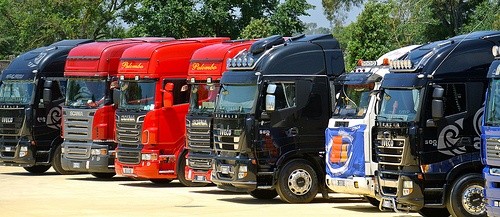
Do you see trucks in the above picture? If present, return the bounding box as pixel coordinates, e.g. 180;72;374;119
184;37;289;193
209;34;345;203
374;31;500;217
479;57;500;217
324;44;421;212
114;37;232;186
59;37;177;180
0;38;122;175
